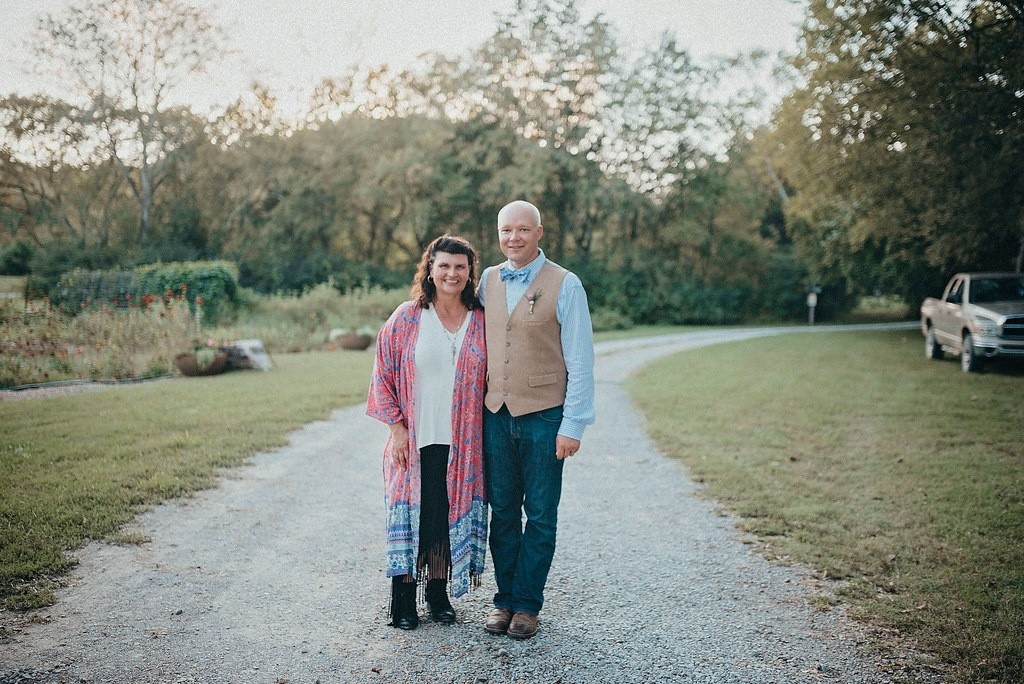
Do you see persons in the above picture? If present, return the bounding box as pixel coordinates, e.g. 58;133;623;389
475;199;595;636
365;235;490;630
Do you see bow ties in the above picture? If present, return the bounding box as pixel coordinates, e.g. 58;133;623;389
500;268;531;283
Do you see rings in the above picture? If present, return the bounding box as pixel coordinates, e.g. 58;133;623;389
393;452;399;457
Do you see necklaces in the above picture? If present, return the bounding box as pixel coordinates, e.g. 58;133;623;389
435;303;465;367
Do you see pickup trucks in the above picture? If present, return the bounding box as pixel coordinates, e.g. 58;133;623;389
919;272;1024;375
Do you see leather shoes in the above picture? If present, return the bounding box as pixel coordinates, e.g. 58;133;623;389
484;608;513;634
507;611;538;638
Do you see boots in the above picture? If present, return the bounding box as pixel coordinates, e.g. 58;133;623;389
391;575;418;629
425;578;456;622
